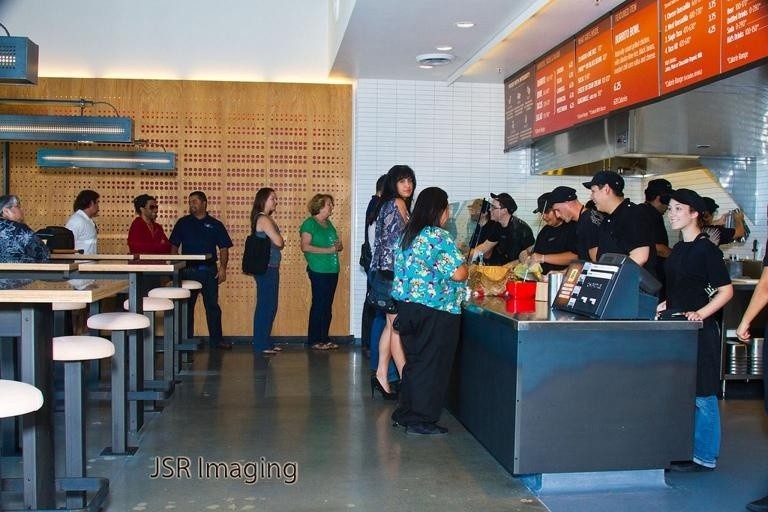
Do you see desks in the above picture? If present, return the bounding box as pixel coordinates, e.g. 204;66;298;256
46;253;137;335
78;261;186;436
139;252;213;364
0;262;79;408
0;279;130;511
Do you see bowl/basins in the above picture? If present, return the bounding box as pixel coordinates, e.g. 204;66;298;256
506;298;535;313
747;336;764;375
506;282;536;298
727;341;747;375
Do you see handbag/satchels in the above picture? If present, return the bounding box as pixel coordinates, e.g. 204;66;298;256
31;227;75;254
366;270;398;314
241;235;271;276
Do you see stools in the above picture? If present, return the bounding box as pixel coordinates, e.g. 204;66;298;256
87;312;150;458
53;337;116;512
166;280;202;363
52;303;87;335
146;287;192;380
0;379;44;419
123;297;175;412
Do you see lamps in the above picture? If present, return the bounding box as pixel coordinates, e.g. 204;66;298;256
0;36;39;85
0;114;135;143
36;147;175;170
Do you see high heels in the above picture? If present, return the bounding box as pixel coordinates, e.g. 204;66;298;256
371;374;399;401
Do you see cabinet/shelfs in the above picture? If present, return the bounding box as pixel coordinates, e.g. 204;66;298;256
715;284;768;400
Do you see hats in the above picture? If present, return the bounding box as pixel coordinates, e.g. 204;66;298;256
659;188;702;213
489;192;518;213
582;171;625;192
532;192;552;214
548;186;577;208
646;178;676;194
699;197;719;211
468;200;488;213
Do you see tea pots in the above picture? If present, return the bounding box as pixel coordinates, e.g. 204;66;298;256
728;208;740;230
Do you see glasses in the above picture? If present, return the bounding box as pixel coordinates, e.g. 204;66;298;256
144;205;158;209
490;203;503;209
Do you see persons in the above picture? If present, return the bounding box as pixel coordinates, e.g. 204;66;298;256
64;190;100;290
169;190;233;350
360;165;744;474
735;203;768;511
250;188;284;354
0;195;50;290
299;194;344;351
127;194;172;314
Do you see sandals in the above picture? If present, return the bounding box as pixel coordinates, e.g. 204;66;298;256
326;342;339;348
312;343;330;350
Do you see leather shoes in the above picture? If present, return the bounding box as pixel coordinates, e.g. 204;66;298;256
273;347;283;351
405;416;449;436
670;461;720;473
262;350;277;353
212;340;232;350
745;495;768;512
